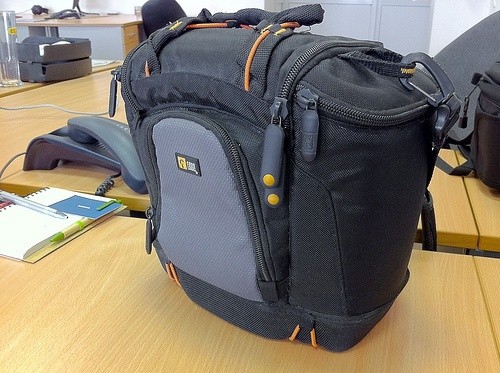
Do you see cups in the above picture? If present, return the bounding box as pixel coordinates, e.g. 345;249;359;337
0;11;21;87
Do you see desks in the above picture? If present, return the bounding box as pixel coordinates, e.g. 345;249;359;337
0;60;500;373
14;15;143;60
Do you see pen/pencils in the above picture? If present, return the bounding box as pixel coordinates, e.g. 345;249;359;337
49;199;122;243
0;190;68;220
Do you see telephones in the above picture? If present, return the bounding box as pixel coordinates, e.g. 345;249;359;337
44;9;80;19
23;113;150;194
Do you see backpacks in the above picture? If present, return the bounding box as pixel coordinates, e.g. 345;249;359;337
435;58;500;191
107;3;463;353
